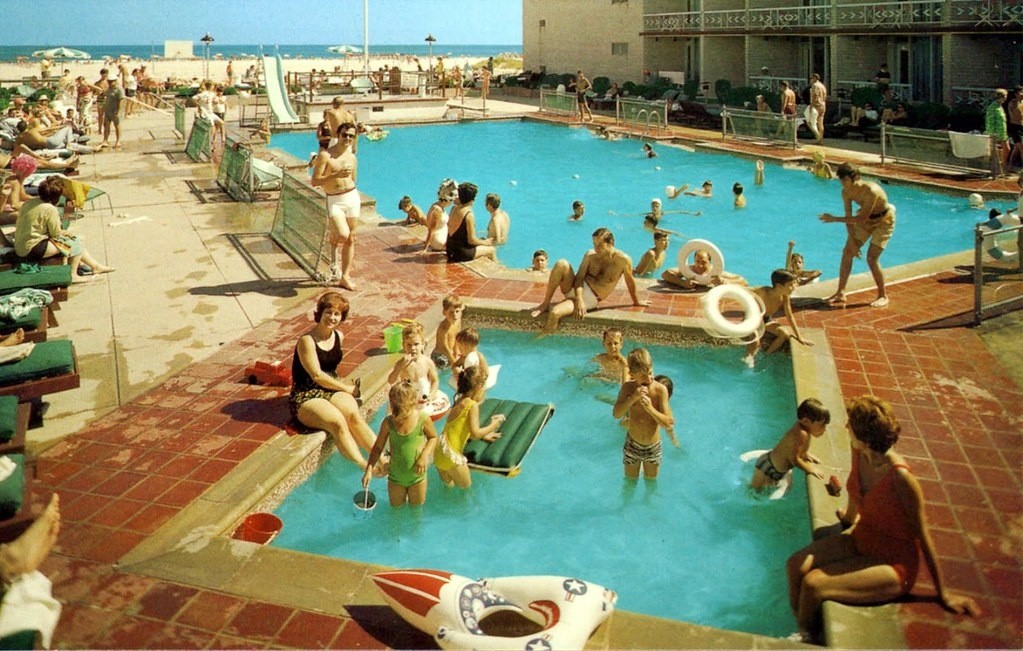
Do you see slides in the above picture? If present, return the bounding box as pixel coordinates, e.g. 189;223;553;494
262;53;301;124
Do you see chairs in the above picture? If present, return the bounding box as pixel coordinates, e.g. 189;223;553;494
503;70;911;143
0;167;80;544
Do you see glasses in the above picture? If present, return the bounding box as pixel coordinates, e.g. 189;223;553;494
340;133;354;138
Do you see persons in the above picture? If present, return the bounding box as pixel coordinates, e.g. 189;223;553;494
0;493;61;592
451;328;489;392
430;295;465;369
612;348;675;477
752;398;830;491
786;394;982;646
362;381;438;506
0;56;271;224
311;124;361;291
388;323;438;418
308;57;910;405
985;84;1023;273
14;176;116;283
433;366;502;488
289;292;391;479
0;328;25;348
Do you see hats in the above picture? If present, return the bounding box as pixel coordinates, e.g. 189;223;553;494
39;95;49;101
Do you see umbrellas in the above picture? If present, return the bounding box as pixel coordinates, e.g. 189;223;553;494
32;46;91;74
327;45;363;63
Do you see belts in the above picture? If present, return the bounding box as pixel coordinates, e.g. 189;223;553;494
870;208;890;219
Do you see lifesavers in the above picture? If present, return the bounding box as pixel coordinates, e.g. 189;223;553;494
701;284;762;337
385;388;451;422
984;214;1020;261
679;239;724;285
367;569;618;650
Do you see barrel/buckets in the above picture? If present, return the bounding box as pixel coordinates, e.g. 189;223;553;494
244;512;283;546
382;326;403;353
353;487;377;516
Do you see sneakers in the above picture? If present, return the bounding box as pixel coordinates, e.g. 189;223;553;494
77;266;92;276
